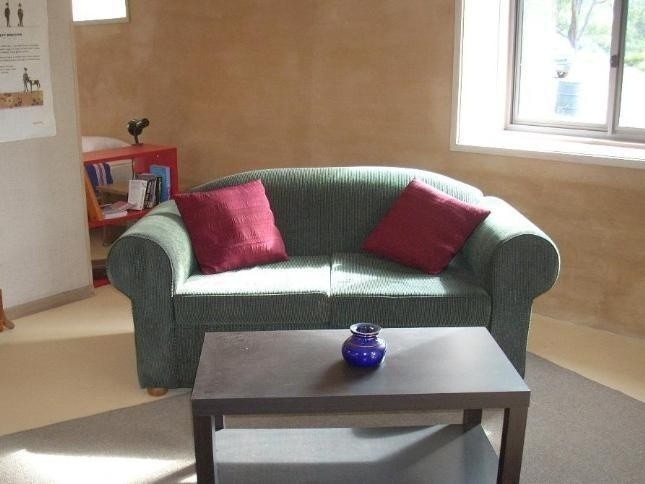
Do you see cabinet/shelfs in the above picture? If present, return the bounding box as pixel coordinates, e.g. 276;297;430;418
83;141;179;288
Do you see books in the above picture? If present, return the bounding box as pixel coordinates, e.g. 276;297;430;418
111;200;129;209
100;205;128;219
125;163;170;210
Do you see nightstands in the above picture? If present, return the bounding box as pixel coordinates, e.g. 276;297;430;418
94;183;132;246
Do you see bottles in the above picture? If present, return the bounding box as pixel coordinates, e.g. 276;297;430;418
341;323;387;367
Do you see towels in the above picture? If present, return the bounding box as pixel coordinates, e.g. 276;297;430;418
85;163;113;185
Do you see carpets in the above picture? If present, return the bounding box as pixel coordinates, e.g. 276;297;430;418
0;349;645;484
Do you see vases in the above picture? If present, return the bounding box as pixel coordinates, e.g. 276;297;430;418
341;322;386;373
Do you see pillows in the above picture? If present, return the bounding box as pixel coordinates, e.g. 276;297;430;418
171;175;290;275
355;177;492;274
82;135;132;166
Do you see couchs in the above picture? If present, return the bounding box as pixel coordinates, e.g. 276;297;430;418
103;166;560;397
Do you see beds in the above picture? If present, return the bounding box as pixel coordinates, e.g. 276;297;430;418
83;157;135;187
